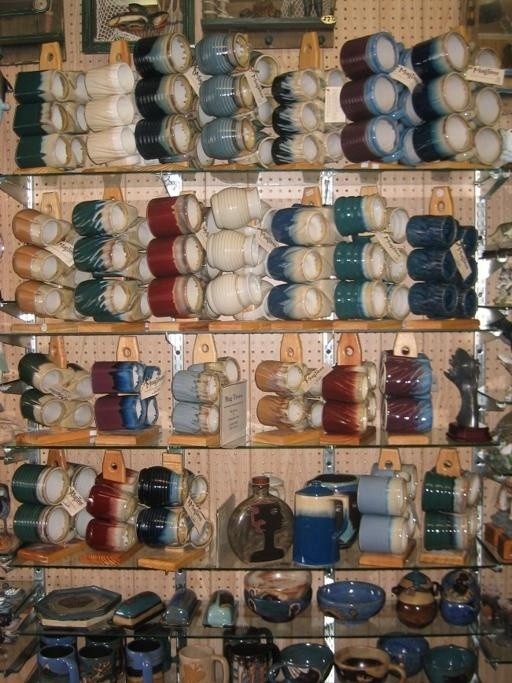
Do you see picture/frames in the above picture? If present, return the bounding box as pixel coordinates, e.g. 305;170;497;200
82;0;195;55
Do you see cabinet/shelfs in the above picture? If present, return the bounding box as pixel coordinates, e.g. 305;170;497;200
1;32;512;683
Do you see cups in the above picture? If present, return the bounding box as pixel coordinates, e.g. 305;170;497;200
453;47;504;166
12;178;484;326
16;564;486;682
12;345;485;442
11;458;483;559
11;27;486;169
